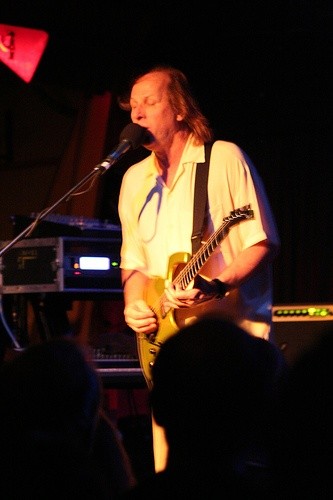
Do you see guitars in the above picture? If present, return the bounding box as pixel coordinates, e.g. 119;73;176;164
136;204;255;399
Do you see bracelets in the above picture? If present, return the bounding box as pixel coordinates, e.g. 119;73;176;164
212;279;227;299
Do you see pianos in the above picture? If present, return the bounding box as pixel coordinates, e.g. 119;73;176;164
272;294;333;323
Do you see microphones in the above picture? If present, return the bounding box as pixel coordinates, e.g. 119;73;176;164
94;123;145;175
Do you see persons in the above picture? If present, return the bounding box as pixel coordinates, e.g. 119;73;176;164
0;324;333;500
117;68;280;473
